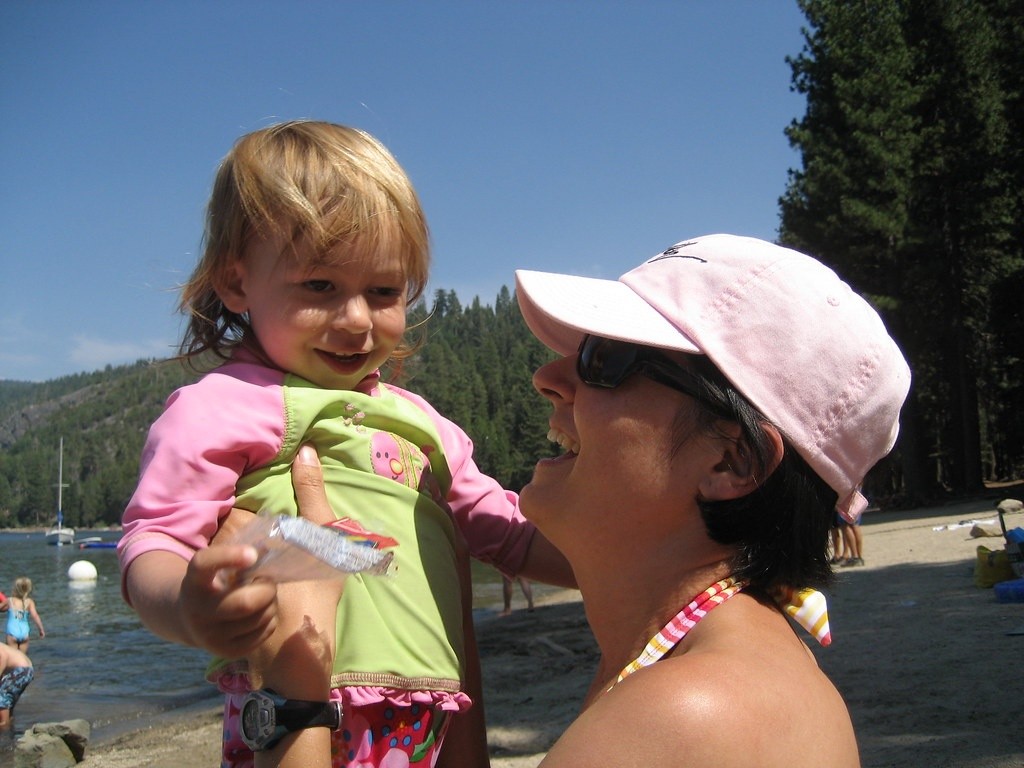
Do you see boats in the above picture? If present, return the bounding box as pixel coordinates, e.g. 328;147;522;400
74;536;117;549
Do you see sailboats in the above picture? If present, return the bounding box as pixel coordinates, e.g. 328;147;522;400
45;437;76;547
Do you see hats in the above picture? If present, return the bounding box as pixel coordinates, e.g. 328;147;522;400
513;235;912;527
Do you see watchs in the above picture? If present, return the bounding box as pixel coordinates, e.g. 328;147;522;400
238;687;344;751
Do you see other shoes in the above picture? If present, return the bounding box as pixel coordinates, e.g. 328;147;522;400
830;555;849;565
840;557;864;567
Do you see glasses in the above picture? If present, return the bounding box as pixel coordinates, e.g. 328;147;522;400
574;330;736;424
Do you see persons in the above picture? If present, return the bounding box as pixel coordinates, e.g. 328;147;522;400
116;118;582;768
210;232;911;768
828;478;865;568
0;641;34;726
0;577;45;654
497;574;534;617
